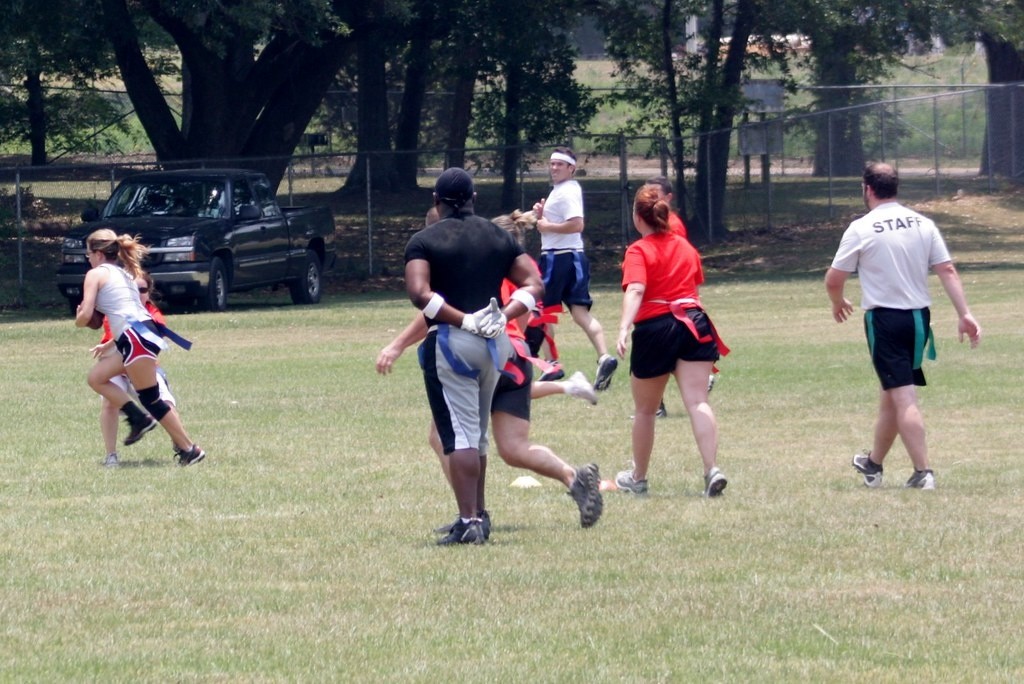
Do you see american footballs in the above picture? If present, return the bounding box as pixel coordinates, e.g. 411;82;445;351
79;301;104;329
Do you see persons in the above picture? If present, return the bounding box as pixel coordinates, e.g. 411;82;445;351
825;161;980;492
617;175;730;495
378;147;617;545
75;229;206;467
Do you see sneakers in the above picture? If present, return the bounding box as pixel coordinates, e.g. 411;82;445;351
904;469;935;491
594;352;619;389
853;454;882;488
180;444;206;467
538;359;565;382
434;509;492;539
568;371;596;406
704;468;727;497
616;469;647;496
567;463;602;529
125;415;157;446
439;519;484;547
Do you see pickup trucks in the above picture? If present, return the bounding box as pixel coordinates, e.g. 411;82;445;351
55;169;335;315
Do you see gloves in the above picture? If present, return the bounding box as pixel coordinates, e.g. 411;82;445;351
479;297;507;336
460;309;505;339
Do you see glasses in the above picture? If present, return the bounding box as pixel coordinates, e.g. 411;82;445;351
85;249;98;258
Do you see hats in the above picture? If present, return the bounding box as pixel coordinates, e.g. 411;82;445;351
434;166;475;207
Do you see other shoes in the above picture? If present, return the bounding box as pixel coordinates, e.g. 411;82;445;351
105;454;118;467
708;375;715;392
655;410;667;418
